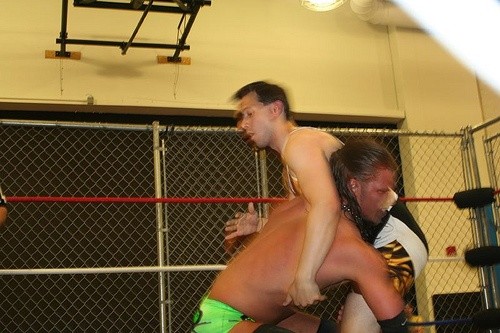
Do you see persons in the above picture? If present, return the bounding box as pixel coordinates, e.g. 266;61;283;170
194;137;408;333
224;82;428;333
403;303;425;333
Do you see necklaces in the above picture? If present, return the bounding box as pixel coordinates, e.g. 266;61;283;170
340;204;358;219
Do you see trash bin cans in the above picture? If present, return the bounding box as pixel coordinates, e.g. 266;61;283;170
432;291;485;333
